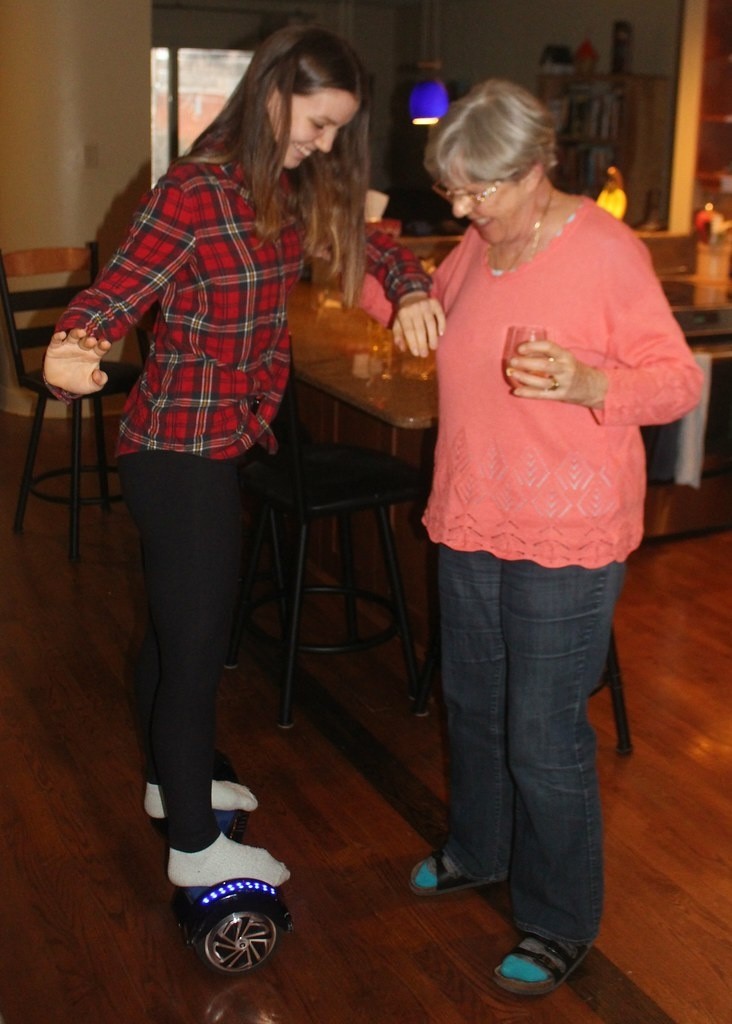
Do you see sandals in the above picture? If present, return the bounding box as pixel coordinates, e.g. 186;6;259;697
408;849;496;896
492;930;594;994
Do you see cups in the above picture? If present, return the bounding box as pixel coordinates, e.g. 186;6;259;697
696;241;732;279
502;326;549;388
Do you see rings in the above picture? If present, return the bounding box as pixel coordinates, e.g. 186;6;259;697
549;376;559;390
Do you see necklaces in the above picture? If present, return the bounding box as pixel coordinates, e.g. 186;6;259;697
483;184;553;279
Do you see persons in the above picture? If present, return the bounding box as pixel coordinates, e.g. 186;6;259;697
42;22;702;994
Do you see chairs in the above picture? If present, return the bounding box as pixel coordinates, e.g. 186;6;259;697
0;240;144;562
224;356;428;730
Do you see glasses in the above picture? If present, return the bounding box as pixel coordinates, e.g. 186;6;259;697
432;180;503;206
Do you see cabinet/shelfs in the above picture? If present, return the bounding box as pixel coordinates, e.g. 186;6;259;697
538;73;648;230
